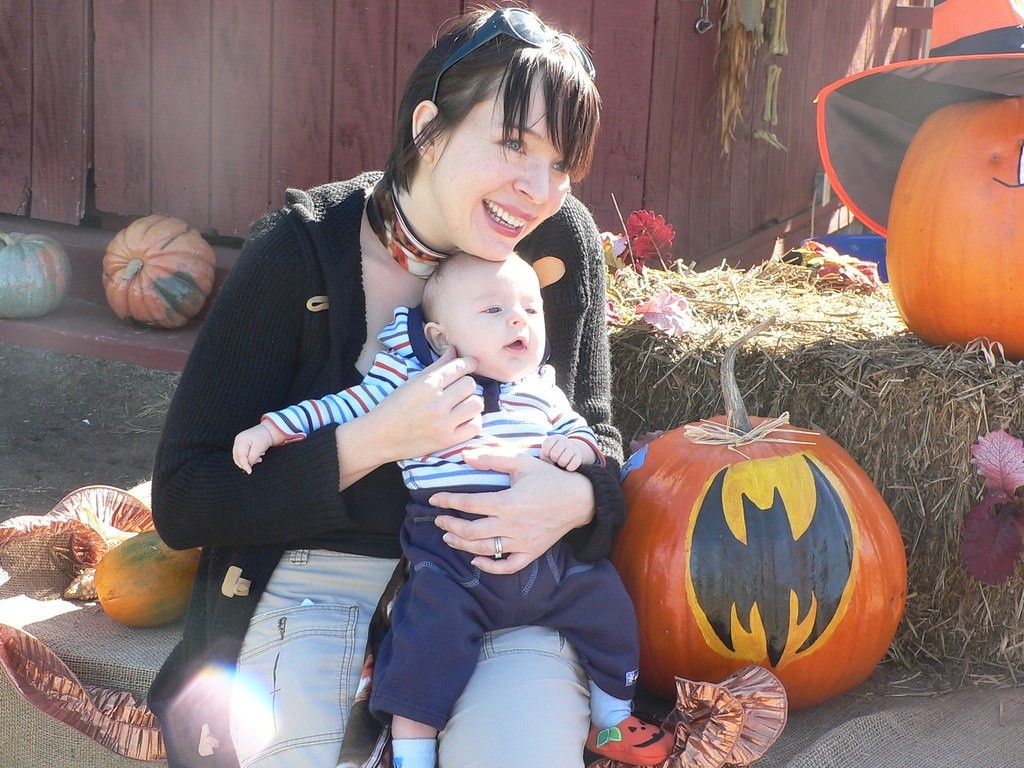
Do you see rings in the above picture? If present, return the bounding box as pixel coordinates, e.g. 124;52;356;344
494;537;503;558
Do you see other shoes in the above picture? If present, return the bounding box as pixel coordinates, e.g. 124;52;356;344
585;715;676;766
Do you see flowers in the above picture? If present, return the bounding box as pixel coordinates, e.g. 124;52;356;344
586;209;696;337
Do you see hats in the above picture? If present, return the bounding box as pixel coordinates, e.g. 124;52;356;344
818;0;1024;238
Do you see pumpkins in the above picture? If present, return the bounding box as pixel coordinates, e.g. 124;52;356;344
93;533;207;625
606;416;907;707
98;216;215;323
1;230;69;317
889;97;1024;360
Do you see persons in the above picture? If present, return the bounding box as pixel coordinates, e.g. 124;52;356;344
230;250;674;768
146;1;623;768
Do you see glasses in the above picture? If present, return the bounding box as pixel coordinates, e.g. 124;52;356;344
432;7;597;104
